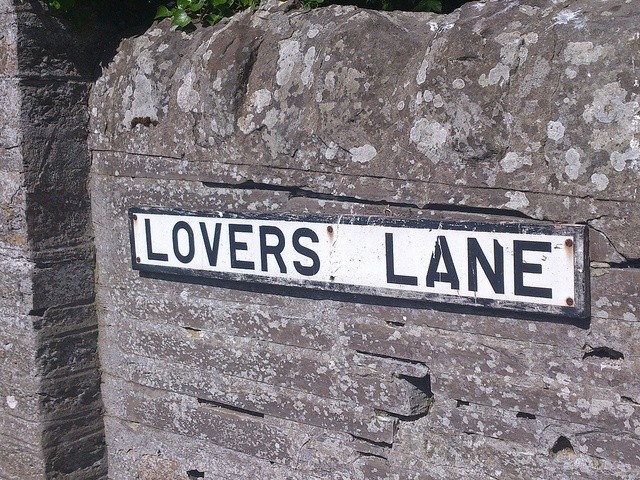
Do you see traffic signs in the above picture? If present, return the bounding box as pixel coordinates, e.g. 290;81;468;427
127;206;591;321
